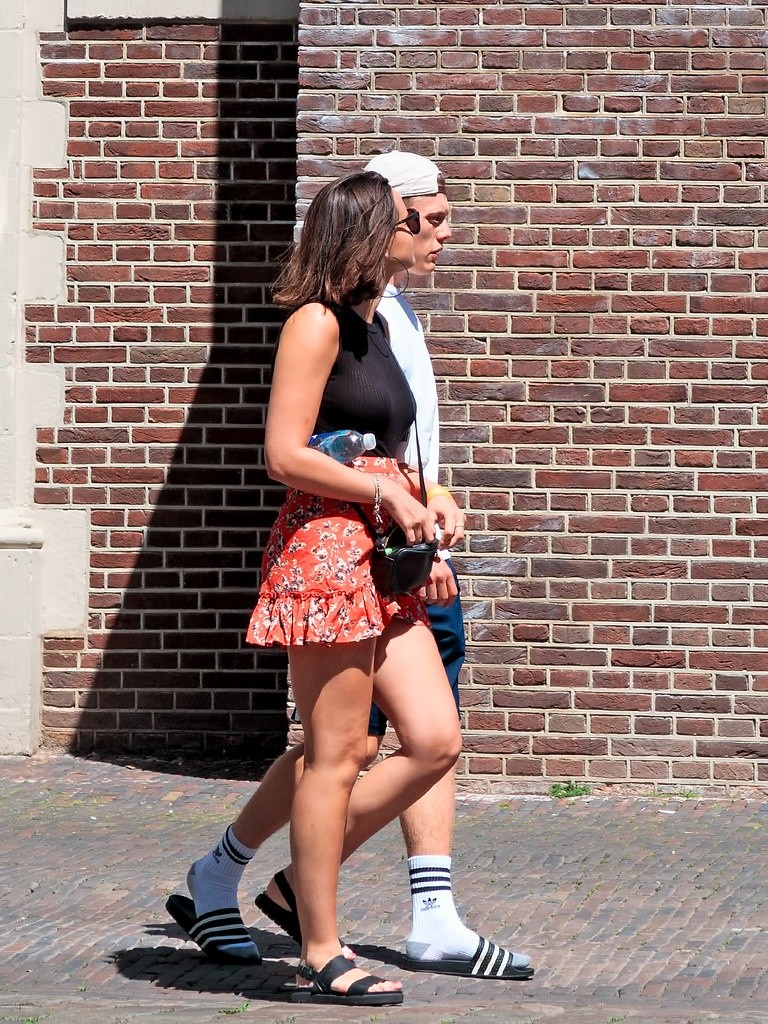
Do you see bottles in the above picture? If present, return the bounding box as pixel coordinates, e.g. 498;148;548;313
307;430;377;466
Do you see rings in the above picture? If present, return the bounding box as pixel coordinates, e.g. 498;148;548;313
456;525;464;528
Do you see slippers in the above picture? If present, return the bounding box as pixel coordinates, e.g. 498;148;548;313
401;934;536;978
165;893;262;966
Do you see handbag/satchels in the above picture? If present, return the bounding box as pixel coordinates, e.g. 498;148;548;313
371;526;437;594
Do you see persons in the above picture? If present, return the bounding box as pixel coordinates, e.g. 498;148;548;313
165;149;535;981
244;169;463;1005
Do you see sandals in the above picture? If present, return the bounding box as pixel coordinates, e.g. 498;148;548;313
255;871;357;965
291;956;404;1005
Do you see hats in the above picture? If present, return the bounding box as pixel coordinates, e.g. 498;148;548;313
362;149;447;197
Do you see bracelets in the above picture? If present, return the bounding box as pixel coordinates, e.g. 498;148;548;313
373;473;383;523
426;486;453;501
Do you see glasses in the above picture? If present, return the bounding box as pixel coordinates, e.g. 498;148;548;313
391;208;421;234
